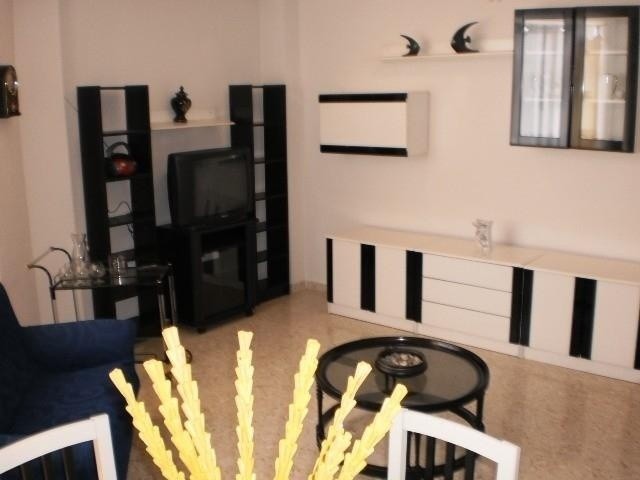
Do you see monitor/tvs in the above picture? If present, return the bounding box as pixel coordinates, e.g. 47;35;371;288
167;148;254;231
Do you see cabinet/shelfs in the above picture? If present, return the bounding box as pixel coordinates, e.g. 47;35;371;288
229;83;289;304
324;237;416;332
25;246;193;379
520;266;639;383
153;217;258;336
415;251;526;357
75;83;162;338
510;4;640;154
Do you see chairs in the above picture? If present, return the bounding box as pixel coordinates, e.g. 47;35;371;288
386;407;521;480
0;283;138;480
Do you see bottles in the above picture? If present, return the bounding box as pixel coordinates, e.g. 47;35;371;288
71;233;89;277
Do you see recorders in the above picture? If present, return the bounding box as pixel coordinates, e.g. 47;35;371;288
103;142;137;178
